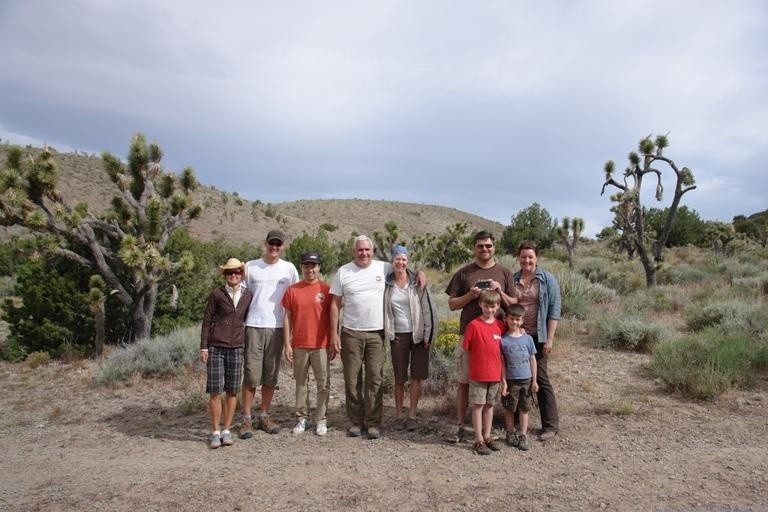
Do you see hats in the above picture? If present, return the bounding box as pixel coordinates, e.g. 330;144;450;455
301;252;322;264
267;230;283;243
220;258;245;275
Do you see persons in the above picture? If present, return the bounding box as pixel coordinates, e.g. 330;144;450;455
328;234;426;440
461;288;527;455
498;303;539;451
280;252;338;436
444;230;519;443
384;244;438;432
511;239;562;441
199;256;253;448
239;230;301;439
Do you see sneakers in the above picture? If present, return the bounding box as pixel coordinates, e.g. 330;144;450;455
259;415;279;434
519;434;529;450
447;424;464;443
239;418;253;439
395;416;407;430
407;417;416;431
350;424;364;436
540;431;558;440
293;419;312;434
507;431;519;447
367;424;380;438
316;419;327;436
473;442;491;455
484;437;502;451
222;434;232;445
211;435;221;448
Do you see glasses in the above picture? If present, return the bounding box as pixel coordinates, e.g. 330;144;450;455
224;270;242;275
475;244;494;249
269;241;282;246
